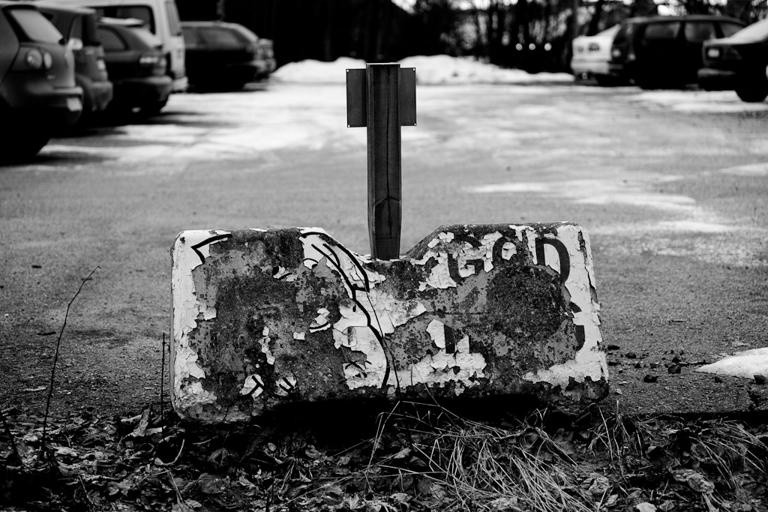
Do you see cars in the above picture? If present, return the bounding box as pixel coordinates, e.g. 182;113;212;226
694;19;768;102
180;20;277;93
569;22;652;87
0;1;188;166
608;13;752;90
